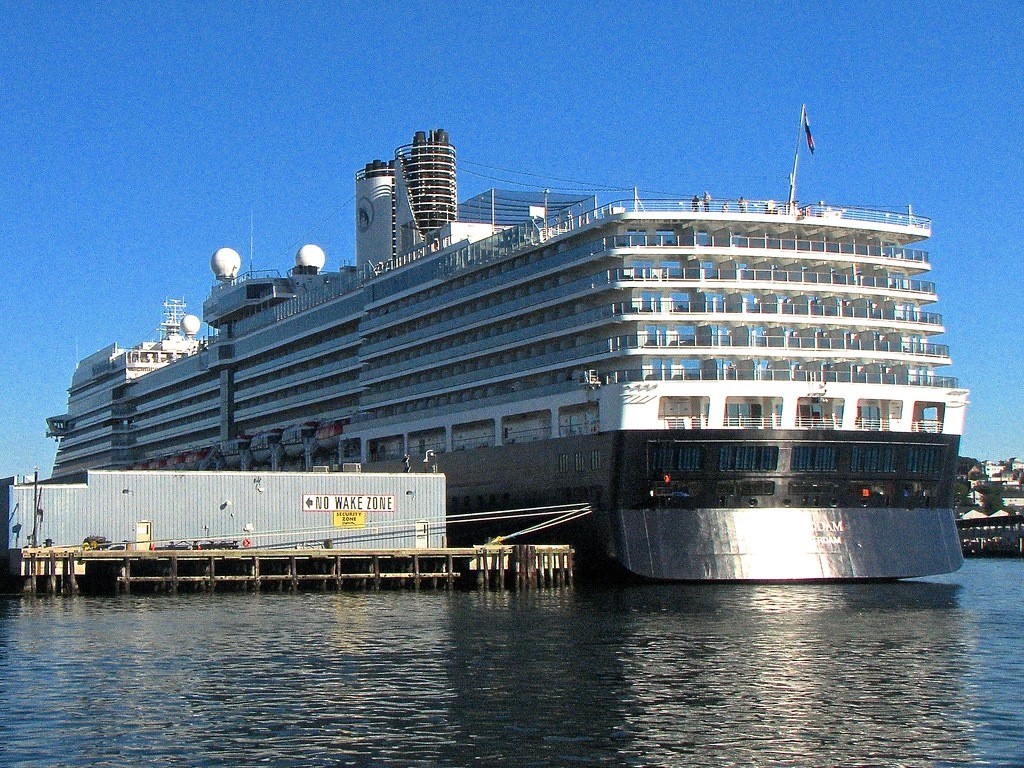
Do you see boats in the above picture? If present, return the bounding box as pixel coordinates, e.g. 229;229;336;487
148;456;168;471
185;447;209;470
250;427;284;462
315;418;347;448
281;423;320;458
221;433;254;466
165;451;188;470
121;465;133;471
132;462;149;471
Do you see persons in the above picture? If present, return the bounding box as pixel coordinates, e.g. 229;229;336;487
703;191;710;212
692;195;700;212
721;202;729;212
738;197;746;213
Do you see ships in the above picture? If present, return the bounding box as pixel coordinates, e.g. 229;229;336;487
48;100;969;586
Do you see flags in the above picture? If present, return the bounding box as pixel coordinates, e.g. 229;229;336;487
801;107;815;155
